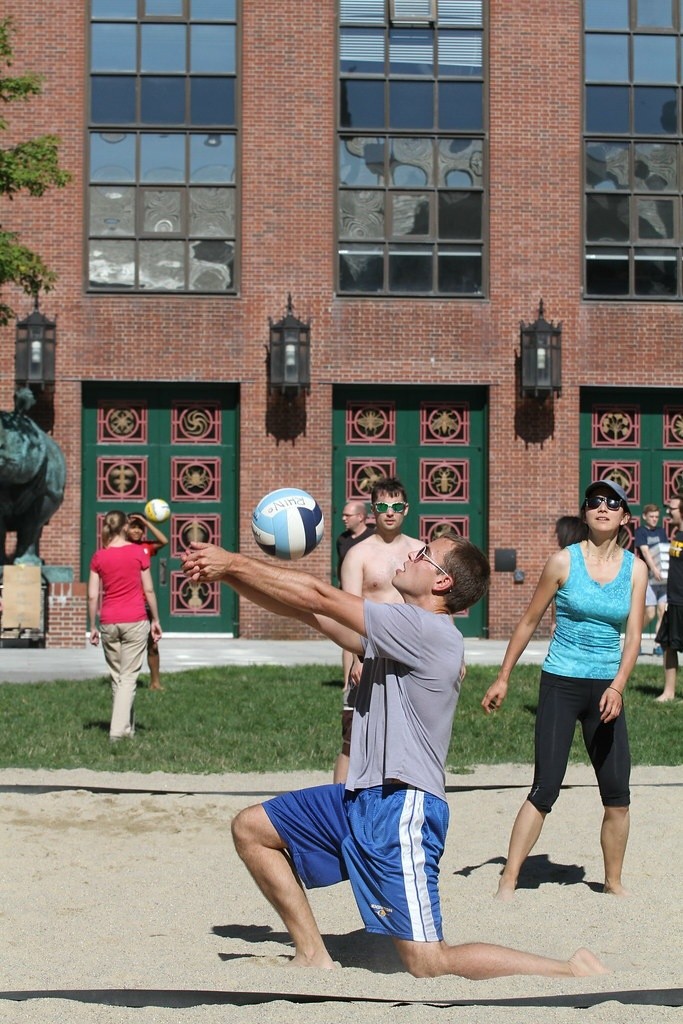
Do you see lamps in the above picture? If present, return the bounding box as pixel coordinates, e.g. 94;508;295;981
519;298;564;405
266;291;312;394
11;297;59;393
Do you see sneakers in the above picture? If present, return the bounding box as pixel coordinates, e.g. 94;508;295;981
653;644;664;658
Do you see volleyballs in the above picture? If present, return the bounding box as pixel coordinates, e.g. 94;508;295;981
251;487;326;561
144;498;171;523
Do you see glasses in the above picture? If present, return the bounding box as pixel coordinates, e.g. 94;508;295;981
374;502;406;513
584;497;623;511
415;546;453;593
342;513;360;519
668;506;679;512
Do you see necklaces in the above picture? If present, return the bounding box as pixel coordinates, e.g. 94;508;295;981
587;544;619;560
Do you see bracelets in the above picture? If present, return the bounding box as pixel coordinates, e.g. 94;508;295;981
609;687;622;696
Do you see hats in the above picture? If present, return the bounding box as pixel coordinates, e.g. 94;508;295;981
581;479;632;516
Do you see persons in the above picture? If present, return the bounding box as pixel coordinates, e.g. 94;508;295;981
179;534;605;981
336;501;377;692
333;477;465;783
88;510;162;740
482;479;648;896
653;495;683;701
126;513;168;688
634;504;667;656
